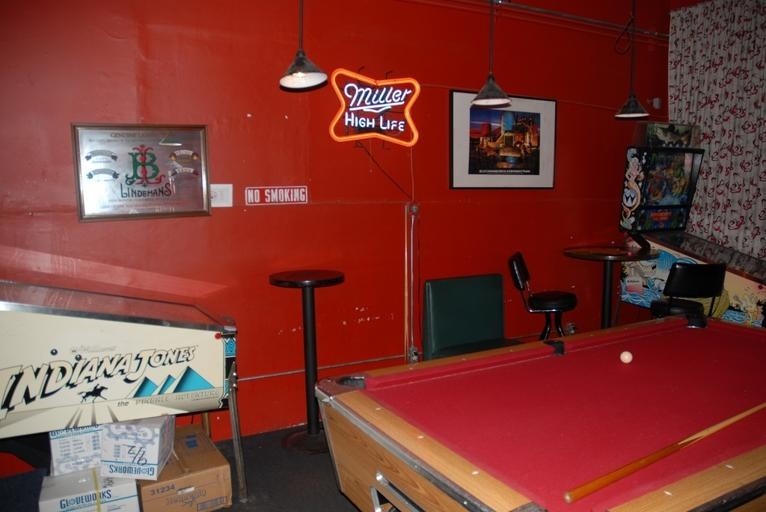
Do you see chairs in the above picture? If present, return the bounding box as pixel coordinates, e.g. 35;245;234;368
649;260;732;330
503;249;579;344
417;271;524;359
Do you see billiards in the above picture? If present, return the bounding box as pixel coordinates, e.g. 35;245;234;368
621;351;633;363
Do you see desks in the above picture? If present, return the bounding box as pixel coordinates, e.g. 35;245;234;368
265;261;344;452
562;234;661;335
312;313;765;512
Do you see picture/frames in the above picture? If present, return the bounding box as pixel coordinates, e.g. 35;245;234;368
73;120;216;224
448;93;562;193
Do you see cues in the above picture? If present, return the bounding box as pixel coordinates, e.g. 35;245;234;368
564;401;766;502
404;205;408;361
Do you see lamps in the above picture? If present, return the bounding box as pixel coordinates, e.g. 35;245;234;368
613;1;653;124
274;1;332;94
469;0;517;110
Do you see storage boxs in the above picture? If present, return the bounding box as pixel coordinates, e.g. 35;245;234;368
34;407;236;512
